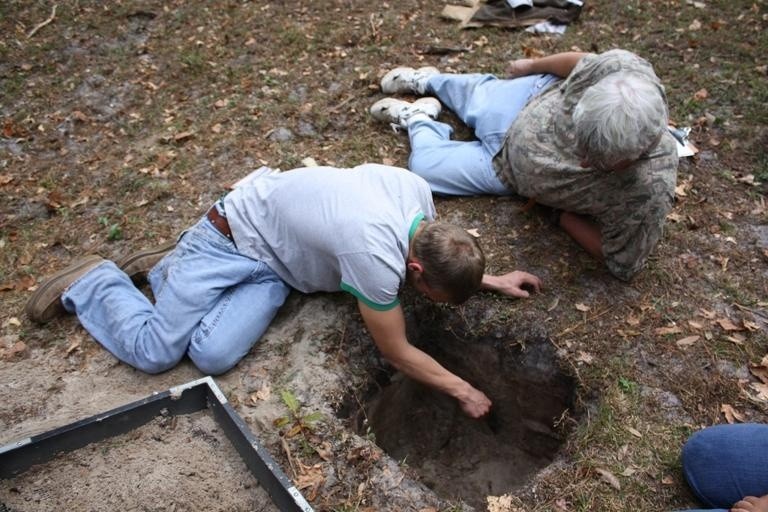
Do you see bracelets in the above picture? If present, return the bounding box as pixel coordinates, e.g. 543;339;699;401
550;208;567;222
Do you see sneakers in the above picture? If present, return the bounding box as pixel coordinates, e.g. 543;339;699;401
117;239;176;284
27;255;104;325
379;65;441;96
370;97;442;128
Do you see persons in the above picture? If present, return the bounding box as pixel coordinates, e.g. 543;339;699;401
681;425;768;512
24;160;546;420
368;49;682;284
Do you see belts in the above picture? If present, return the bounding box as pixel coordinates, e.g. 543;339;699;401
207;205;232;243
